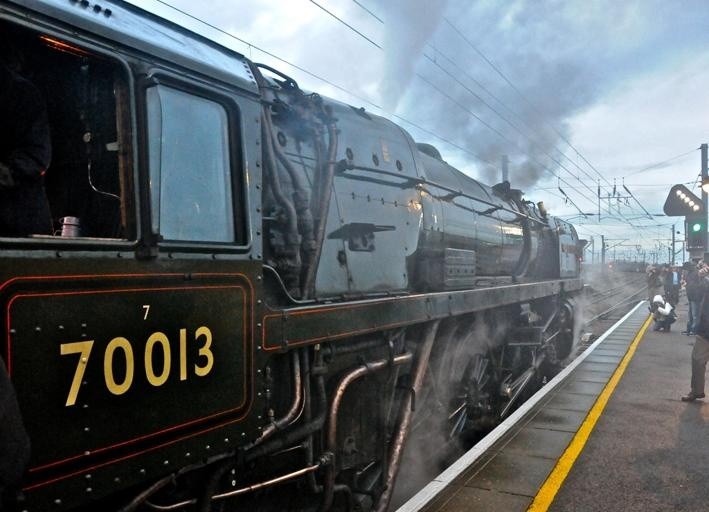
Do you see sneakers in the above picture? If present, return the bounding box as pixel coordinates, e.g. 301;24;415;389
682;391;706;403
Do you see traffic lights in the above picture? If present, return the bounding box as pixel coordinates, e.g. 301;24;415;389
687;219;706;249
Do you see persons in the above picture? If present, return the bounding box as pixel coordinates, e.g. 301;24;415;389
681;265;709;402
645;256;709;335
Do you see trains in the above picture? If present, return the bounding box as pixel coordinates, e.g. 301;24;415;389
0;0;588;510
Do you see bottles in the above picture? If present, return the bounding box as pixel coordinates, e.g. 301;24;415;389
60;216;79;237
